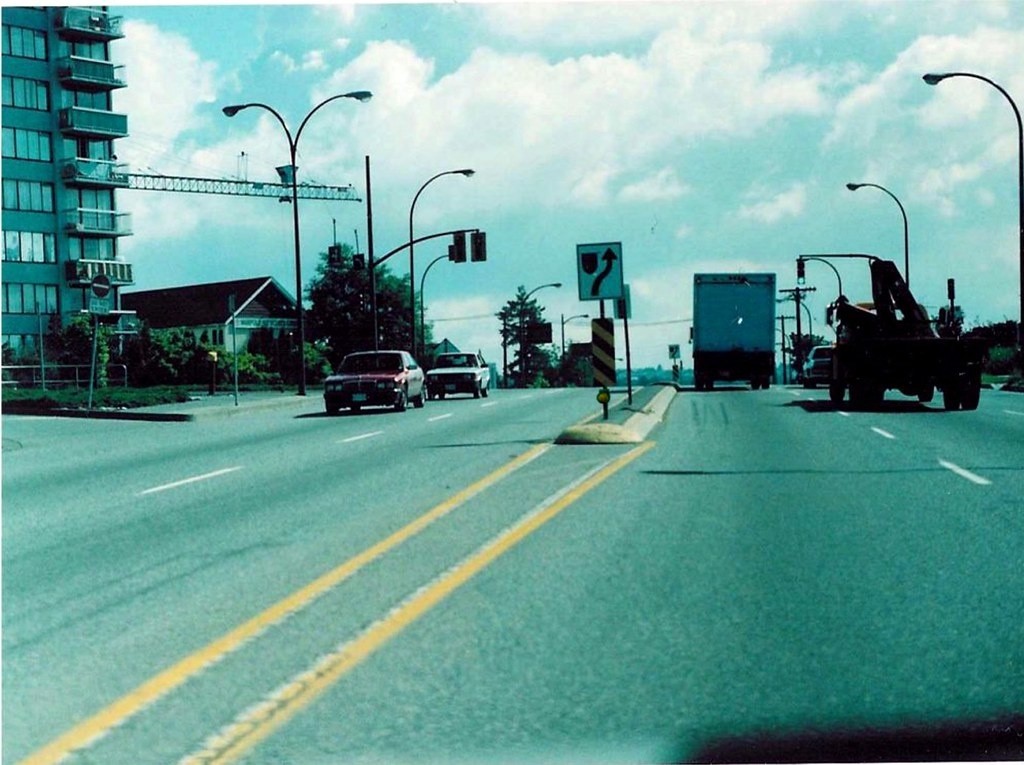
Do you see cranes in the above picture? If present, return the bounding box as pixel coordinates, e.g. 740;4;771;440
112;165;361;203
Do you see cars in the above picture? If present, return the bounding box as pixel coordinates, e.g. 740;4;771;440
424;353;491;399
801;345;836;389
325;349;426;412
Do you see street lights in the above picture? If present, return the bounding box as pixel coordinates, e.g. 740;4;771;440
562;314;589;386
846;182;909;290
777;299;812;340
221;90;374;394
923;71;1024;351
519;282;564;389
410;168;478;355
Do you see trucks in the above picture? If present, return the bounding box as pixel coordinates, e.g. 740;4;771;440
689;273;776;388
829;302;989;412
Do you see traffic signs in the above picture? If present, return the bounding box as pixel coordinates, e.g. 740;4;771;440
579;243;622;300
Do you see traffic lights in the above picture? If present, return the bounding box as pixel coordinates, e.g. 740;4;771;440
797;261;805;285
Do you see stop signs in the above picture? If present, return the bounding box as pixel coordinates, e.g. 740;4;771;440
92;274;110;297
